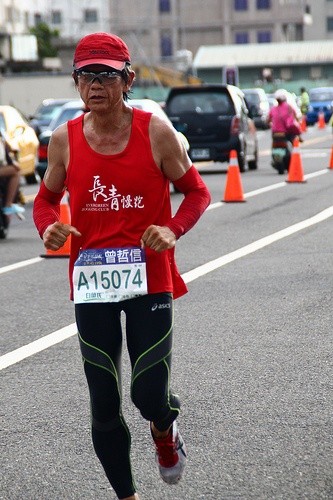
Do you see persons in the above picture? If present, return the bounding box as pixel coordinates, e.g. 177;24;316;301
34;31;210;500
299;87;310;117
0;128;27;220
265;88;297;144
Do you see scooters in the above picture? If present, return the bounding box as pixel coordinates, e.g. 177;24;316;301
271;130;290;174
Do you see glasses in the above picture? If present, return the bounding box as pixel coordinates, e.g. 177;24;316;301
76;71;123;86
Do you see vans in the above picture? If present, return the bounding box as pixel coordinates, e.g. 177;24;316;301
161;84;259;175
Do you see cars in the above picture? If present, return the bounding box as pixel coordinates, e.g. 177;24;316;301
0;106;41;184
301;85;333;123
242;88;273;130
264;94;278;108
26;98;75;135
36;100;190;188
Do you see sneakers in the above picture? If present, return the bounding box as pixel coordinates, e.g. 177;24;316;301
150;421;189;485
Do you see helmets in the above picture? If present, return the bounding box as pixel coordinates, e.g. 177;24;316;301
273;88;288;101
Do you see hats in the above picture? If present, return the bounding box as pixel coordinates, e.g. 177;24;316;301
73;32;131;71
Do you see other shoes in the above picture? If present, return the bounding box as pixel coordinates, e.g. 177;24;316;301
2;203;26;216
16;212;25;221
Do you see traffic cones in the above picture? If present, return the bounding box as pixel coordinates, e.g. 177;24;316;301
318;112;326;128
219;149;249;202
36;188;73;258
327;143;333;170
300;115;306;130
283;135;309;183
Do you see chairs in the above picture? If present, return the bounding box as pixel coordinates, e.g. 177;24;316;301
213;100;225;111
